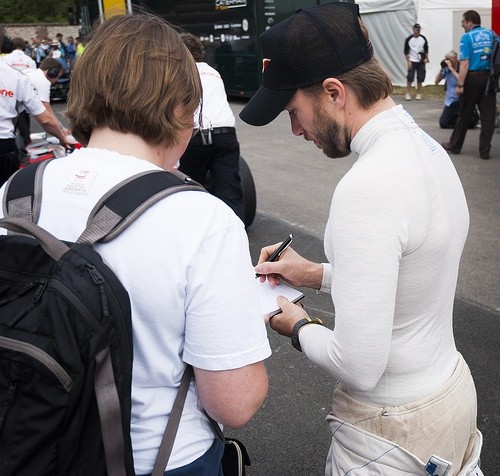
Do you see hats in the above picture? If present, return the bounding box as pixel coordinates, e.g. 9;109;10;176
238;3;375;126
413;23;421;29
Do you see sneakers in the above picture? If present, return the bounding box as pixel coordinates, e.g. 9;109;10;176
405;92;411;101
415;93;422;100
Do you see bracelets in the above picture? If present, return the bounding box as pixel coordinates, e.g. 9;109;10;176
457;83;464;88
291;317;324;352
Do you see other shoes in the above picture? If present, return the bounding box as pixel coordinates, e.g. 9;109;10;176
480;152;490;159
442;142;461;154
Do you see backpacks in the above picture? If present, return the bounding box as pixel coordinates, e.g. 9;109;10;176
490;36;500;92
0;156;210;476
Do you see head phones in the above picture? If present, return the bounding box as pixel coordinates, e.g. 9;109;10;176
47;63;60;78
1;37;17;53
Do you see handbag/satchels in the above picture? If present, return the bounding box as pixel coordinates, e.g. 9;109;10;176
222;436;249;476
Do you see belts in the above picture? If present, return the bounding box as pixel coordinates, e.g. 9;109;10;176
192;128;236;135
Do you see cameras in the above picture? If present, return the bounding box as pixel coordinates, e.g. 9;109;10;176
441;58;449;68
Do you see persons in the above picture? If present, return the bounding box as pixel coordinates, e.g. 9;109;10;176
402;23;429;101
434;9;500;159
0;13;271;476
174;32;242;220
0;24;97;186
237;2;483;476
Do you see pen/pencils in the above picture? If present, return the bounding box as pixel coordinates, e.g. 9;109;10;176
250;233;294;280
